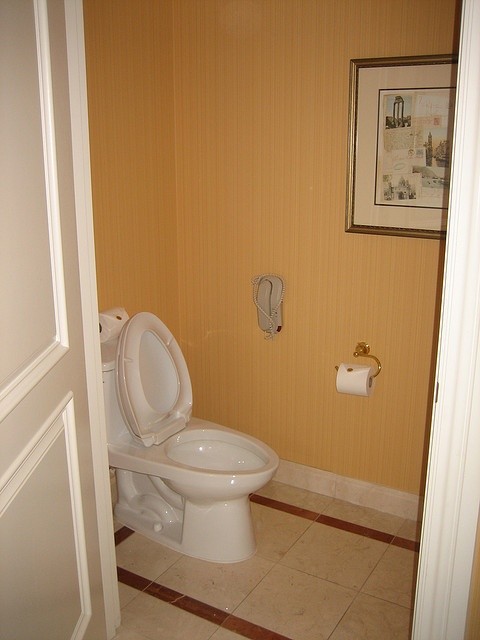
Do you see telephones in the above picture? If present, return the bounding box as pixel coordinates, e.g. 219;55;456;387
251;274;284;340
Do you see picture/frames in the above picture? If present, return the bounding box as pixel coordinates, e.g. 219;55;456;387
344;51;461;238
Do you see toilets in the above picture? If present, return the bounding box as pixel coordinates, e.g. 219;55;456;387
96;311;279;563
